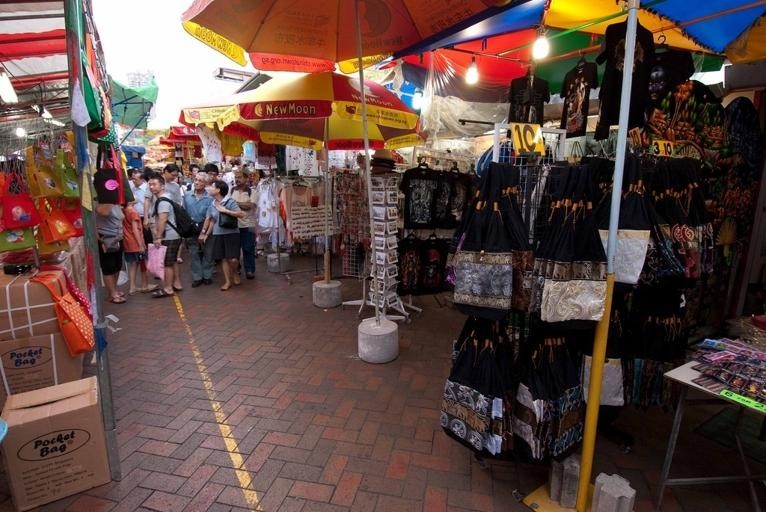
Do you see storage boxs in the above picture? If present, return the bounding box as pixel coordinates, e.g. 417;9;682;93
1;375;112;512
0;236;92;396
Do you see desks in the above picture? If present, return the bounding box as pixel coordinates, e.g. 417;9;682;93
651;359;765;511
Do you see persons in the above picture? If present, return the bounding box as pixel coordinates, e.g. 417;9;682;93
120;161;259;298
95;198;126;304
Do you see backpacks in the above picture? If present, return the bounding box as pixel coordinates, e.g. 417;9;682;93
155;197;197;238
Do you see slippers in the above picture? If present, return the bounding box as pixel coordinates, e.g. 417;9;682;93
107;256;255;304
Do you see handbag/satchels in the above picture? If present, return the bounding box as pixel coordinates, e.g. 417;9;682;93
83;31;135;204
54;291;96;357
439;156;717;461
68;282;93;323
219;212;238;230
0;168;85;262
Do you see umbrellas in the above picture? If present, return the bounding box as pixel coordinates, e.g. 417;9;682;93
180;0;531;326
213;71;428;286
